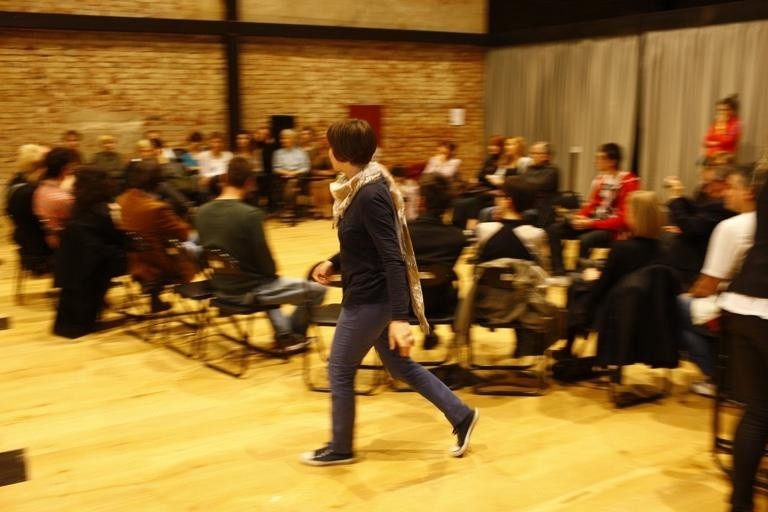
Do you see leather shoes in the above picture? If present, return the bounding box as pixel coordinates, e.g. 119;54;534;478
689;380;718;397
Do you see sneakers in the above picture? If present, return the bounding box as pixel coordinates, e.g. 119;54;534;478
276;333;319;354
448;406;480;458
298;444;358;467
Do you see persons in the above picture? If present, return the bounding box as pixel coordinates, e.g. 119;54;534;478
113;157;201;311
197;157;327;352
390;137;768;403
717;154;767;511
53;164;142;286
704;91;742;168
301;116;479;465
59;124;326;225
32;145;78;266
3;144;57;276
